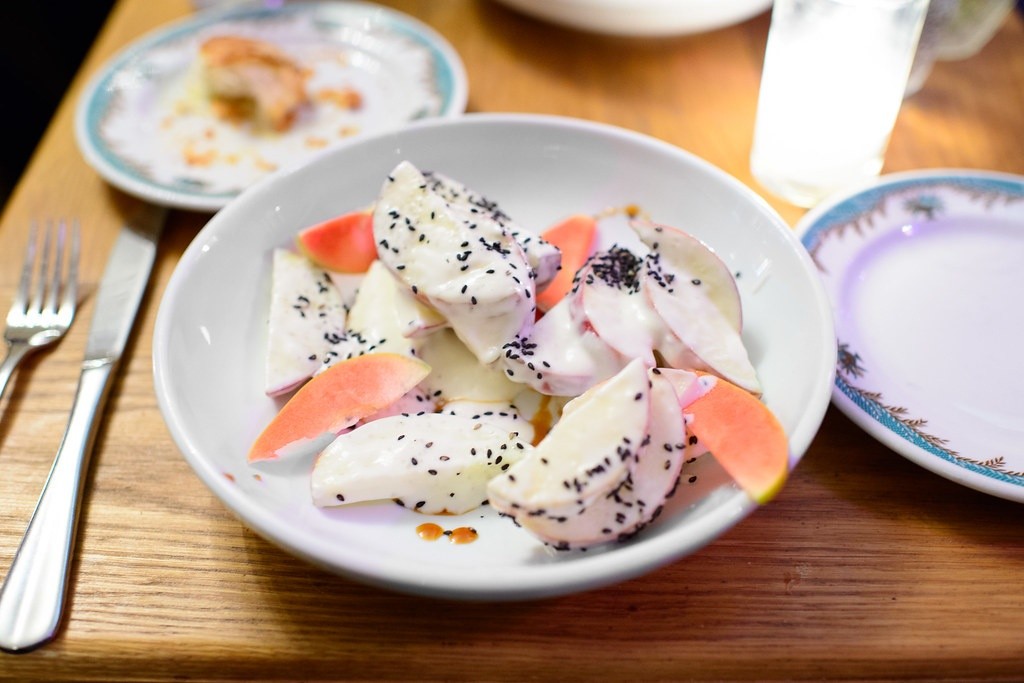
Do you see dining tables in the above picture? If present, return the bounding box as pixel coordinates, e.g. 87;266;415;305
1;0;1024;683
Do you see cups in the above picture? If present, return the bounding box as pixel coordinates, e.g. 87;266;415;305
748;0;932;209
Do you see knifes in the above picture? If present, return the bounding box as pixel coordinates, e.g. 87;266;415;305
0;192;170;653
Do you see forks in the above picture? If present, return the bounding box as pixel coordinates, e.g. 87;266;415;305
0;216;85;404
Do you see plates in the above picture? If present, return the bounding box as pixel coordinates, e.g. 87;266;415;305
148;110;837;602
495;0;774;41
71;0;470;212
790;169;1024;505
